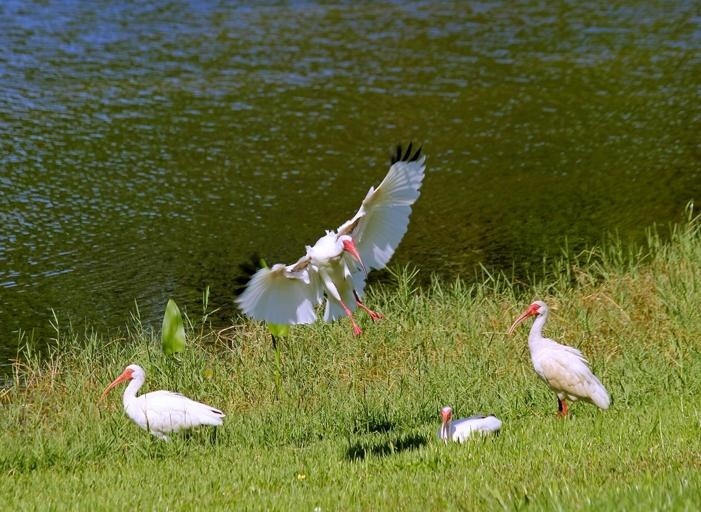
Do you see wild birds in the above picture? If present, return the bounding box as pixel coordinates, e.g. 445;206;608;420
103;364;227;444
435;406;503;446
228;138;428;336
508;300;612;418
